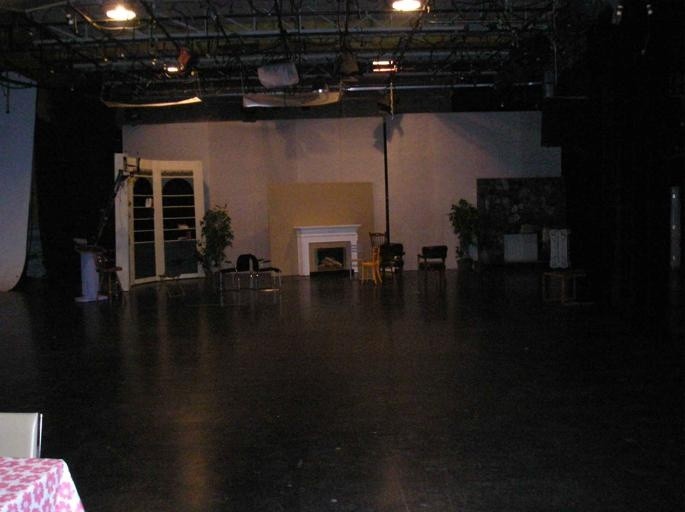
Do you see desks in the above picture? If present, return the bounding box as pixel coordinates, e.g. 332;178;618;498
0;454;85;512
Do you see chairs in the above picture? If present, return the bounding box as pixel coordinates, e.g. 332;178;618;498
159;261;185;298
0;412;43;460
251;254;281;288
219;254;252;292
350;232;405;285
417;246;447;281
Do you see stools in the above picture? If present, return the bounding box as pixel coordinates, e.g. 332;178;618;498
95;268;126;308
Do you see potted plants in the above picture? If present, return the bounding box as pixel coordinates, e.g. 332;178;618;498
446;199;481;274
